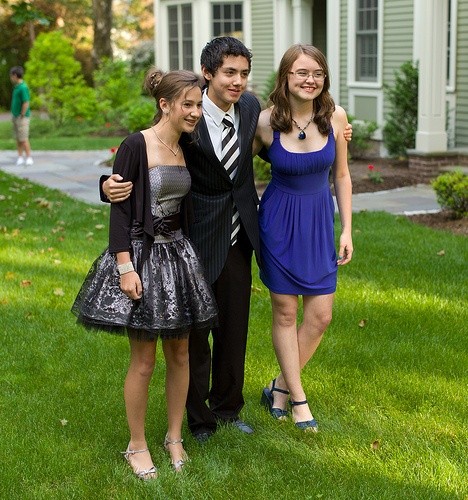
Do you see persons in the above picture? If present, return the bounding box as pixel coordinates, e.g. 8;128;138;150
99;36;353;444
10;66;35;167
252;44;354;435
70;68;221;482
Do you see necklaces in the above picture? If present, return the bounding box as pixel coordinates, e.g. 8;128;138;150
152;127;179;156
292;113;313;140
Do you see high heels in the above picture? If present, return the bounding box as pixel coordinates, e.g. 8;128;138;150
260;378;319;434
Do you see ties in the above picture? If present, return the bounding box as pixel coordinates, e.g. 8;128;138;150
221;114;240;247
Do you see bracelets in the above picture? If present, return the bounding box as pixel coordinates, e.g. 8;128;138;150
117;261;134;275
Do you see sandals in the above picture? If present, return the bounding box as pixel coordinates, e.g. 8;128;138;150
120;441;159;482
163;431;193;472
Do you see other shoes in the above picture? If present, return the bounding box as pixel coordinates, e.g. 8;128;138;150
230;418;253;434
15;157;34;165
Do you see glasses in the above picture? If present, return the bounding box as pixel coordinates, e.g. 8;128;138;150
287;70;327;79
